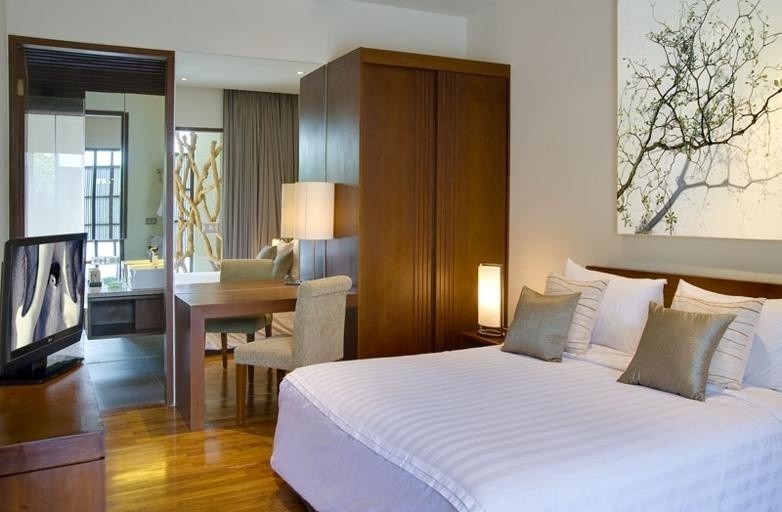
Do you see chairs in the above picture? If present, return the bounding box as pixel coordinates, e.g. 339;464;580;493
232;274;353;427
204;258;274;385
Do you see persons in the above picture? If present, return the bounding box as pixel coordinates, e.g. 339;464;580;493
12;238;82;351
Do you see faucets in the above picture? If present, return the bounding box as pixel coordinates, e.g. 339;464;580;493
146;245;159;262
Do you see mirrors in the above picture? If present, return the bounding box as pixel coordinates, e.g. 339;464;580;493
172;50;319;404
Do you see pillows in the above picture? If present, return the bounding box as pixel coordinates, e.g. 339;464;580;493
254;245;293;281
500;259;770;401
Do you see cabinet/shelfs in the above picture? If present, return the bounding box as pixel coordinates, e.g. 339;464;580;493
327;46;509;361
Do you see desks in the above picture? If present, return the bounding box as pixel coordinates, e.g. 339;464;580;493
173;284;359;433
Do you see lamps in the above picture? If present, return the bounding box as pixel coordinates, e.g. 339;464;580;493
474;264;506;339
281;183;302;286
293;181;335;282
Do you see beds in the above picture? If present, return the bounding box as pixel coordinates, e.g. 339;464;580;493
271;264;781;512
174;240;299;356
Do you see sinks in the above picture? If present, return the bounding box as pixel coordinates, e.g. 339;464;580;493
121;259;165;290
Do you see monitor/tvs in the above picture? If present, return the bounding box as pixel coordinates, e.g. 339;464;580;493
0;232;87;386
